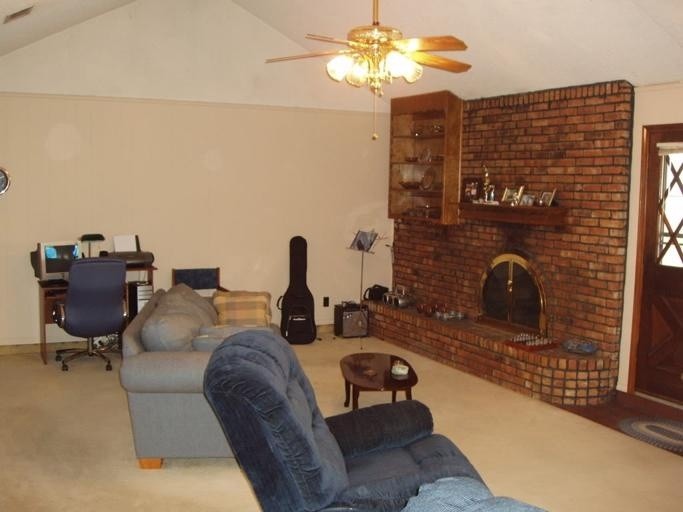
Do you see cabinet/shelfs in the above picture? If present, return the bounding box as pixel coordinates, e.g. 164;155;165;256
386;90;463;226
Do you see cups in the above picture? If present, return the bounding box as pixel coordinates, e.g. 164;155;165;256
416;301;464;322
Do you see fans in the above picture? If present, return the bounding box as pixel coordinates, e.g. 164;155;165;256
264;1;473;141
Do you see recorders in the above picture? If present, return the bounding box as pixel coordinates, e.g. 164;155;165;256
382;285;410;307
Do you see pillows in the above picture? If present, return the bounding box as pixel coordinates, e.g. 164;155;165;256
192;289;271;354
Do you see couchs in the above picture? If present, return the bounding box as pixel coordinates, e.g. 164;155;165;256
119;281;281;469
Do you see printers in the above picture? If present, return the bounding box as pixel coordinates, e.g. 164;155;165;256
99;235;154;269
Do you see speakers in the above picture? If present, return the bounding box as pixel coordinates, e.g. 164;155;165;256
332;304;369;338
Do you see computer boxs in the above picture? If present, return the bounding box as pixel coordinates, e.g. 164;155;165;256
128;281;153;323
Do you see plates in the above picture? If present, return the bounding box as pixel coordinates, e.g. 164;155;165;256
421;168;435;189
421;148;432;164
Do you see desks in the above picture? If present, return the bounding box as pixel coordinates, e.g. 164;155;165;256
36;262;158;364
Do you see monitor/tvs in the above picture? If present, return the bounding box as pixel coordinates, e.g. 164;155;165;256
31;241;77;283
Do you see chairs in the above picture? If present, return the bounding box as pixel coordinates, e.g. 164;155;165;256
203;328;488;511
51;257;127;372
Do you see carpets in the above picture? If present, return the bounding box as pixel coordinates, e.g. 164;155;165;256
617;414;682;452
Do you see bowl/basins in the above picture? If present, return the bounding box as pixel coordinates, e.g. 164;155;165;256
400;181;421;189
403;150;419;162
407;120;444;137
